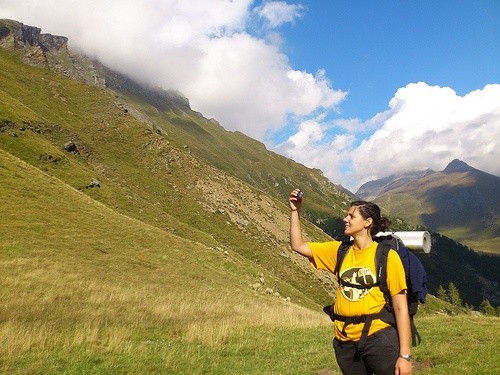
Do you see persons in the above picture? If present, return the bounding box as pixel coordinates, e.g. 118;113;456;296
289;189;413;375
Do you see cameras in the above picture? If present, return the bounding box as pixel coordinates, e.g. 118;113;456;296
296;191;303;199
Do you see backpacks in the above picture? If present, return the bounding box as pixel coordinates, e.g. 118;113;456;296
333;235;430;324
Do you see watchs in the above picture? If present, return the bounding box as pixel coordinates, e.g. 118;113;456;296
398;354;412;361
